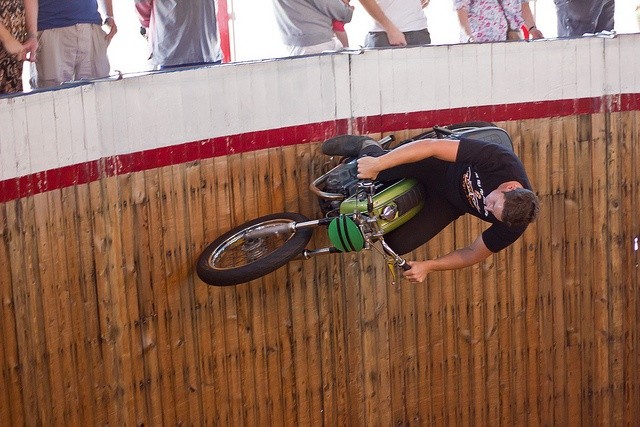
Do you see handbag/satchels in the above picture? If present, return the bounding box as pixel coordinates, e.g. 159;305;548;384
507;29;520;40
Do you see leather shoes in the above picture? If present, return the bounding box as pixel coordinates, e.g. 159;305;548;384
322;135;373;157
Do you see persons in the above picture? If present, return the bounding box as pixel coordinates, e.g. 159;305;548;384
134;0;225;71
271;0;355;57
320;134;540;284
21;0;118;90
359;0;431;46
0;0;27;96
332;0;350;47
553;0;615;38
453;0;545;42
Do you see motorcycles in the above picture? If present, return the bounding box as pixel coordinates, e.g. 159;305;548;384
196;122;514;287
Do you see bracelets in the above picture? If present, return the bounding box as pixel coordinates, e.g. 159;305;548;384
105;15;114;18
529;26;536;31
